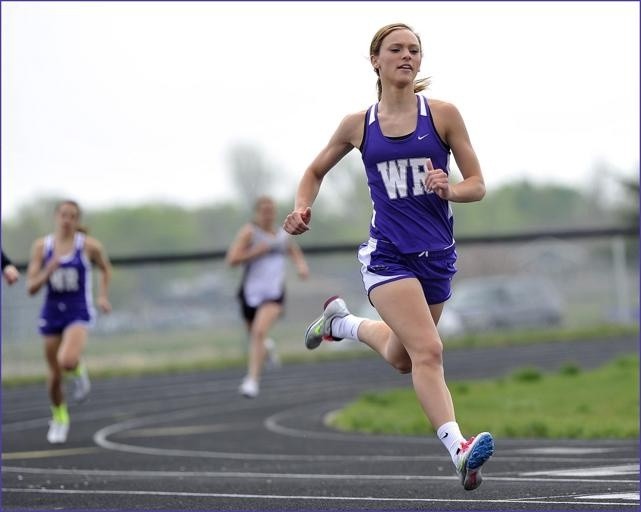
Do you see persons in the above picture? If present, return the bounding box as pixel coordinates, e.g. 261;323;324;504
225;195;310;398
283;23;495;490
1;247;22;286
28;201;112;444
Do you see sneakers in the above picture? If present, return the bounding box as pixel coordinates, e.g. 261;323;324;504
46;418;70;444
239;375;260;401
74;365;91;403
304;294;350;351
455;432;495;491
265;337;281;369
